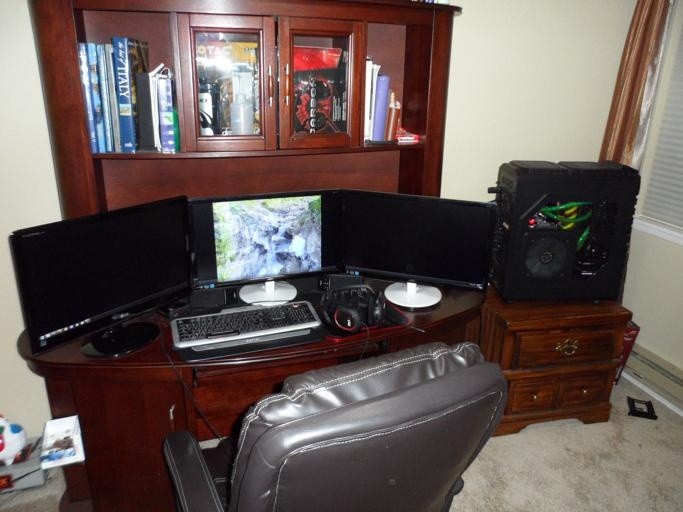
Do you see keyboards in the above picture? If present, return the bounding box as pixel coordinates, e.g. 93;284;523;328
169;301;322;352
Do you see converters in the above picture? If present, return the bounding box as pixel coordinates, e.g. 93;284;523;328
189;286;238;307
319;272;364;291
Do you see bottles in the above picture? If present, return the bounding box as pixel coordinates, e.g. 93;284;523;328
231;92;253;136
198;75;220;136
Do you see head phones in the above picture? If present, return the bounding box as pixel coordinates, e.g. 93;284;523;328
320;284;386;334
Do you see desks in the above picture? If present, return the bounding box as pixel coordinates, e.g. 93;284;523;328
17;276;487;511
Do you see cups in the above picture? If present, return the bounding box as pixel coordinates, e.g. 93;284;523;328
385;107;400;142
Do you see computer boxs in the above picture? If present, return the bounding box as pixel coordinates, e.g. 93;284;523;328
490;160;641;304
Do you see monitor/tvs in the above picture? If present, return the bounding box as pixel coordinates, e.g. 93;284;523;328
10;194;192;358
188;188;342;307
341;189;497;309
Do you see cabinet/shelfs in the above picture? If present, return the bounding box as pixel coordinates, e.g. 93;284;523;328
27;1;462;221
483;288;633;436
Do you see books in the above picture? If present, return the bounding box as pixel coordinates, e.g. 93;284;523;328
365;57;419;144
78;35;182;155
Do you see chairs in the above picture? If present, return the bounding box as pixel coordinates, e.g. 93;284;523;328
163;341;507;512
